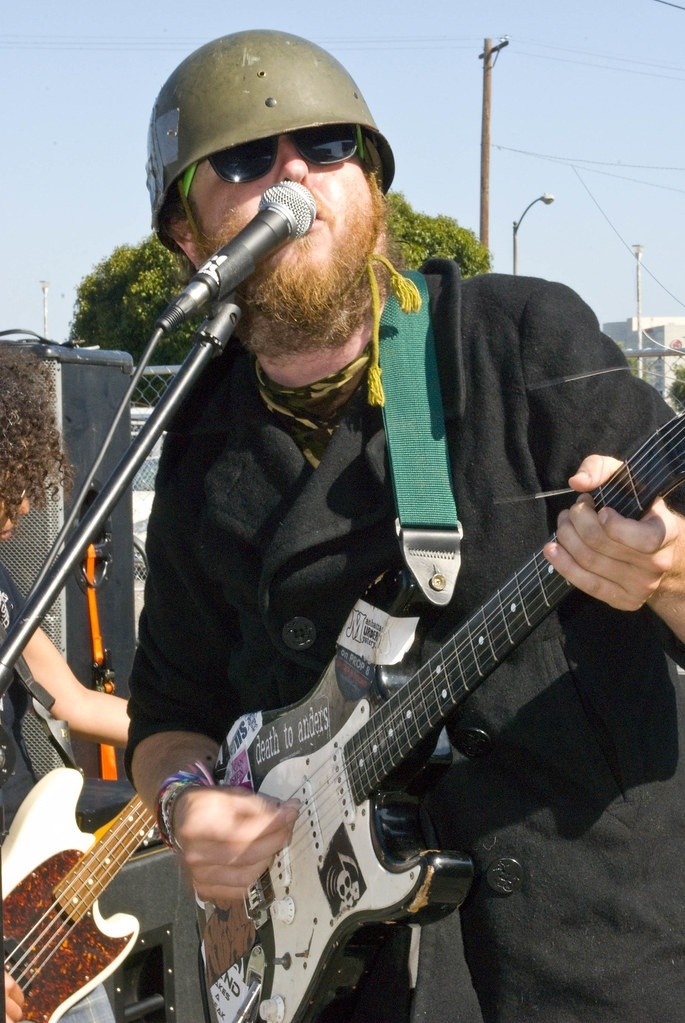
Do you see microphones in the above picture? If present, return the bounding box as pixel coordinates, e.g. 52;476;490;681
153;181;316;330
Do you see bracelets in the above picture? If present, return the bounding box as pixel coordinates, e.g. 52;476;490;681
153;762;217;852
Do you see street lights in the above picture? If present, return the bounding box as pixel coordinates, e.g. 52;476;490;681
513;192;555;274
630;242;646;381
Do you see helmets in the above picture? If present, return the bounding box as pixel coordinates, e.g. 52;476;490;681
145;28;394;256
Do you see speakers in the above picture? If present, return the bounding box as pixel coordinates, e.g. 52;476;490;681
0;330;137;799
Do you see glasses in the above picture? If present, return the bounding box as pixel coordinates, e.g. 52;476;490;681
198;123;358;184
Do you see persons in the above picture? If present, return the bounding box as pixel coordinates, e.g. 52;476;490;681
0;342;131;1023
124;29;684;1023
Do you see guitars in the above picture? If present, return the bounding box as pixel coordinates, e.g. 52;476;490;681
197;409;685;1022
0;765;162;1023
